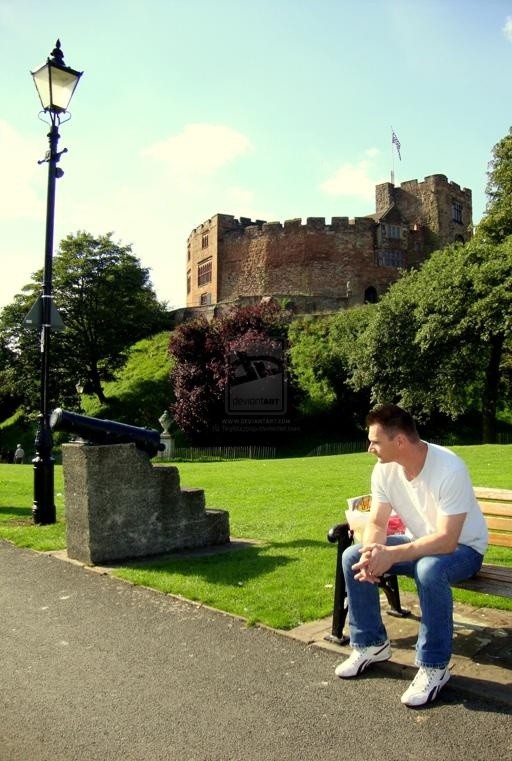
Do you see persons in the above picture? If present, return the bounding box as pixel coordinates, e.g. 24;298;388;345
335;404;488;707
15;444;24;464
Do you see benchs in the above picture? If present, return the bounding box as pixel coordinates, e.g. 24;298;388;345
324;487;512;645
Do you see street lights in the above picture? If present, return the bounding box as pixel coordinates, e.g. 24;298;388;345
27;38;85;528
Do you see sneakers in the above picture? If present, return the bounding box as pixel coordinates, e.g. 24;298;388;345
334;638;392;678
400;664;451;706
